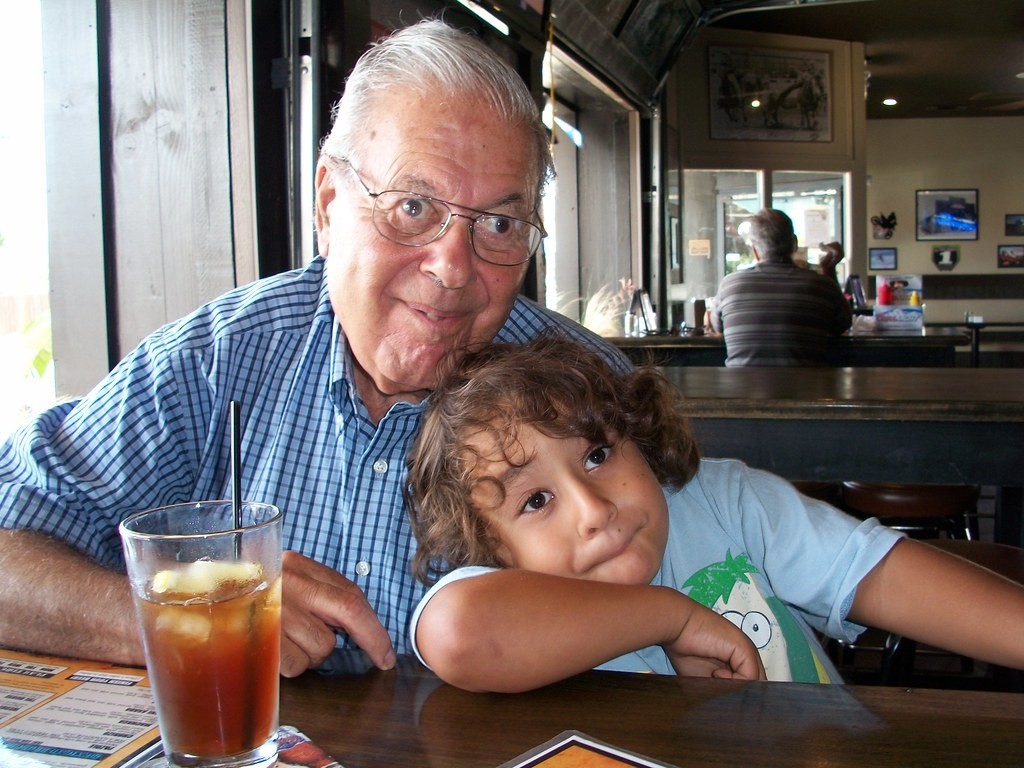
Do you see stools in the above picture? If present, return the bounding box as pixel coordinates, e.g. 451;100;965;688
786;478;982;677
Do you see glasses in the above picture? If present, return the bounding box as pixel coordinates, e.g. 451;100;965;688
348;162;548;266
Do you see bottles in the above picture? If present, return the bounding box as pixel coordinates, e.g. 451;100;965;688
909;289;921;306
878;278;893;305
624;309;646;336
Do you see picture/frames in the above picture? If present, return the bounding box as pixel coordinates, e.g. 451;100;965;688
705;44;834;144
915;189;979;242
868;249;898;270
1004;213;1024;236
997;244;1024;269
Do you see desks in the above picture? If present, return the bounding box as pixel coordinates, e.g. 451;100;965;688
0;644;1024;768
600;327;972;367
628;365;1024;550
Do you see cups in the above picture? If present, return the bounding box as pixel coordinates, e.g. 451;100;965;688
119;500;282;768
938;249;952;264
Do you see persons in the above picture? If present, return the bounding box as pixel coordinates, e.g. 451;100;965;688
709;208;853;369
401;331;1023;680
0;7;642;694
